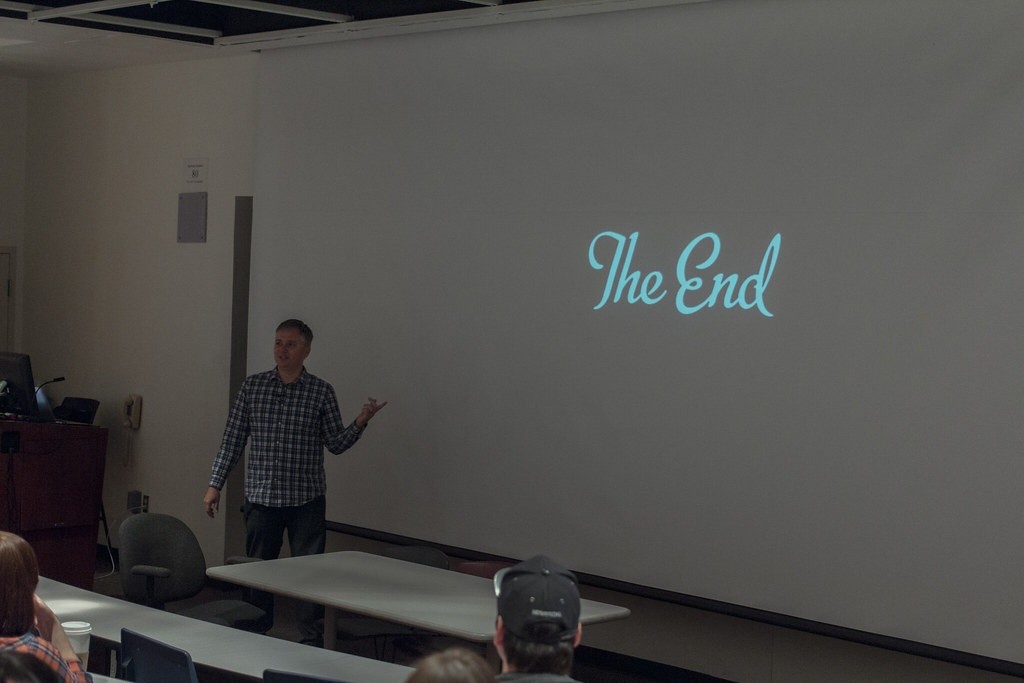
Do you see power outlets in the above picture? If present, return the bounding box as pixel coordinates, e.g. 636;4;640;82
142;495;149;513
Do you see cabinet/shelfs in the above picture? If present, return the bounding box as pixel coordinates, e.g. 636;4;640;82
0;420;110;588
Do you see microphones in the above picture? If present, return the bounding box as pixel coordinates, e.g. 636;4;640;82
34;376;66;394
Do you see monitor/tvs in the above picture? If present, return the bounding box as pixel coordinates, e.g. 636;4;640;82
0;352;36;416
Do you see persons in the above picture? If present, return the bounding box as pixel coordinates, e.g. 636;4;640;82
0;530;94;683
494;555;582;683
406;646;496;683
203;319;387;648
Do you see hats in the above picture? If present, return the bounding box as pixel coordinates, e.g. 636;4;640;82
493;554;583;644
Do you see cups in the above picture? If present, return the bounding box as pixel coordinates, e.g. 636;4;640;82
61;620;92;673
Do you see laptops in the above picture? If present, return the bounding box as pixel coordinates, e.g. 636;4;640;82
55;397;100;424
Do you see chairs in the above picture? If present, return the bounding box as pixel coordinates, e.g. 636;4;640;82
119;509;274;630
391;560;524;666
120;628;198;683
314;546;452;659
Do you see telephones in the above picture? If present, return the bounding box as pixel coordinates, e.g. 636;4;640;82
122;394;143;429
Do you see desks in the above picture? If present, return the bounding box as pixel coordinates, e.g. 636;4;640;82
205;551;632;669
39;574;430;683
